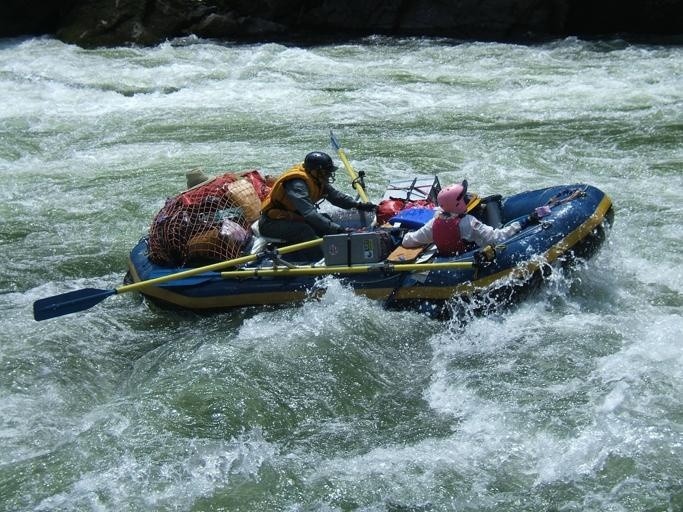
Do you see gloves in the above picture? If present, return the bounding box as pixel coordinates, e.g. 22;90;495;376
337;226;352;233
397;228;408;240
519;212;541;230
356;202;377;212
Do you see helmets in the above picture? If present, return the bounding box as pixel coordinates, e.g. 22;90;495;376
437;179;470;214
304;151;338;172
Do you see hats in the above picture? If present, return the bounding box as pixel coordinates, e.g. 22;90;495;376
180;167;216;194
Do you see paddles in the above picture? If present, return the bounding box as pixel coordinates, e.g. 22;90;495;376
33;237;323;322
329;131;373;204
147;261;488;289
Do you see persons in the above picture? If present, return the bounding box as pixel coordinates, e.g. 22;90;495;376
257;151;379;262
390;184;551;257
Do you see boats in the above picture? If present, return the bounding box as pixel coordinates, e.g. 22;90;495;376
120;180;614;321
